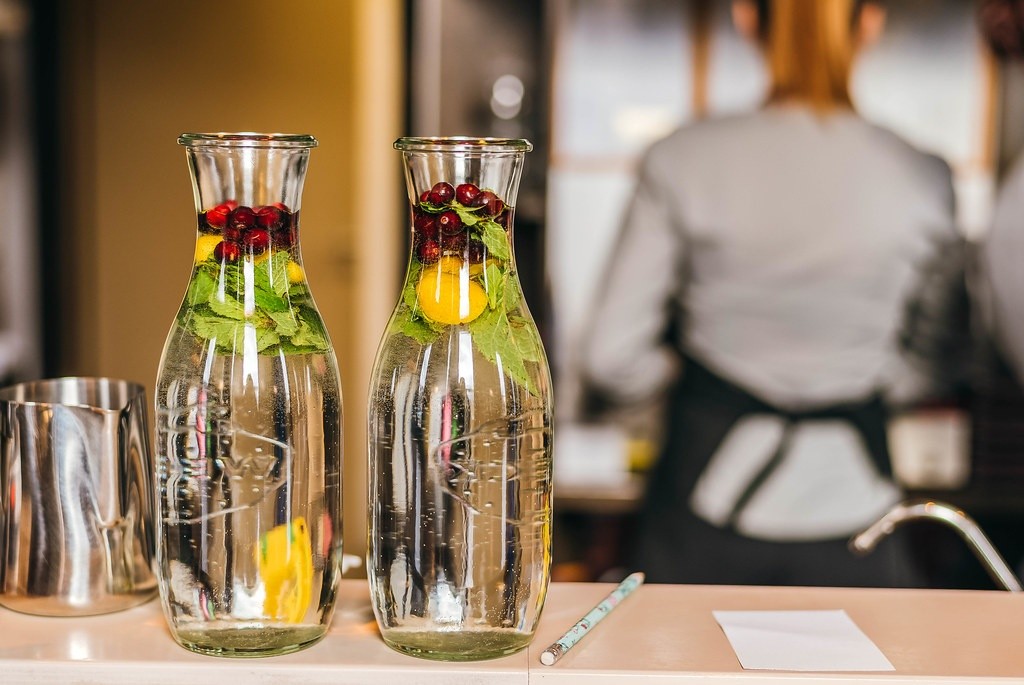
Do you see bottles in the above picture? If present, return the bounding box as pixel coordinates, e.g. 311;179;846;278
152;131;344;658
367;135;555;663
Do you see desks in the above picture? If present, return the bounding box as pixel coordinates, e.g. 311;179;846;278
1;576;1023;678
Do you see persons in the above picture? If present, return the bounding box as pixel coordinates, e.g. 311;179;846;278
574;0;974;587
965;0;1024;591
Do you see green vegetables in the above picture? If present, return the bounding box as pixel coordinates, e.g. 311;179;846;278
173;244;333;357
386;196;545;397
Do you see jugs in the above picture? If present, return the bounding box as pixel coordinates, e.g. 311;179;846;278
0;376;159;619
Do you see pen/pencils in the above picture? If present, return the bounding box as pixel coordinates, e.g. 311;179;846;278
539;571;645;667
